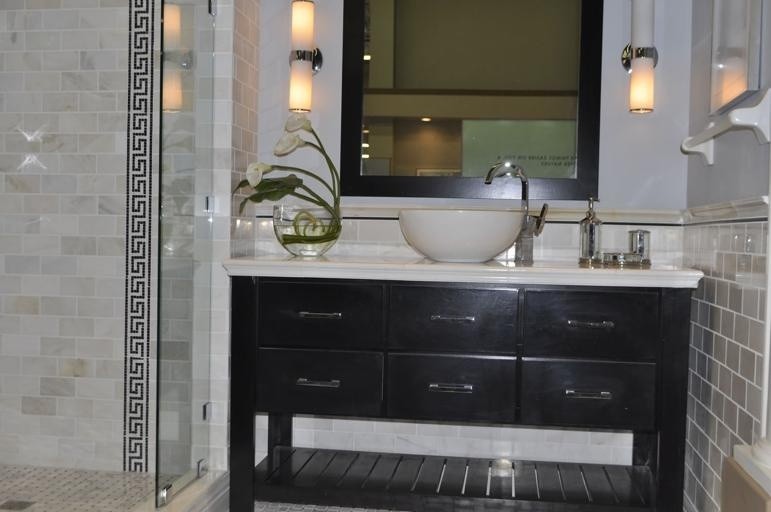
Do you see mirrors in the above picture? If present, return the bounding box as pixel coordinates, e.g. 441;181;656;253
340;0;604;200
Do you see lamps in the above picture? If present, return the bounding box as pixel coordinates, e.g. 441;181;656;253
622;0;657;114
716;0;750;106
288;0;322;113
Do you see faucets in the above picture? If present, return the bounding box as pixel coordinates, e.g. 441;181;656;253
483;163;529;215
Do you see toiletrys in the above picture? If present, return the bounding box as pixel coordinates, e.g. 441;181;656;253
580;198;601;258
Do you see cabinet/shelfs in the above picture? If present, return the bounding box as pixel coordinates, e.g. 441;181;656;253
230;275;695;512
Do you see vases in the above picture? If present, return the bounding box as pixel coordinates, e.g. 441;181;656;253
272;203;339;257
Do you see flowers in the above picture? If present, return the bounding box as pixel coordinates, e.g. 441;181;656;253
233;112;342;244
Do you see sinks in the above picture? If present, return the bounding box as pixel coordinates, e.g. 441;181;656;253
398;208;525;264
